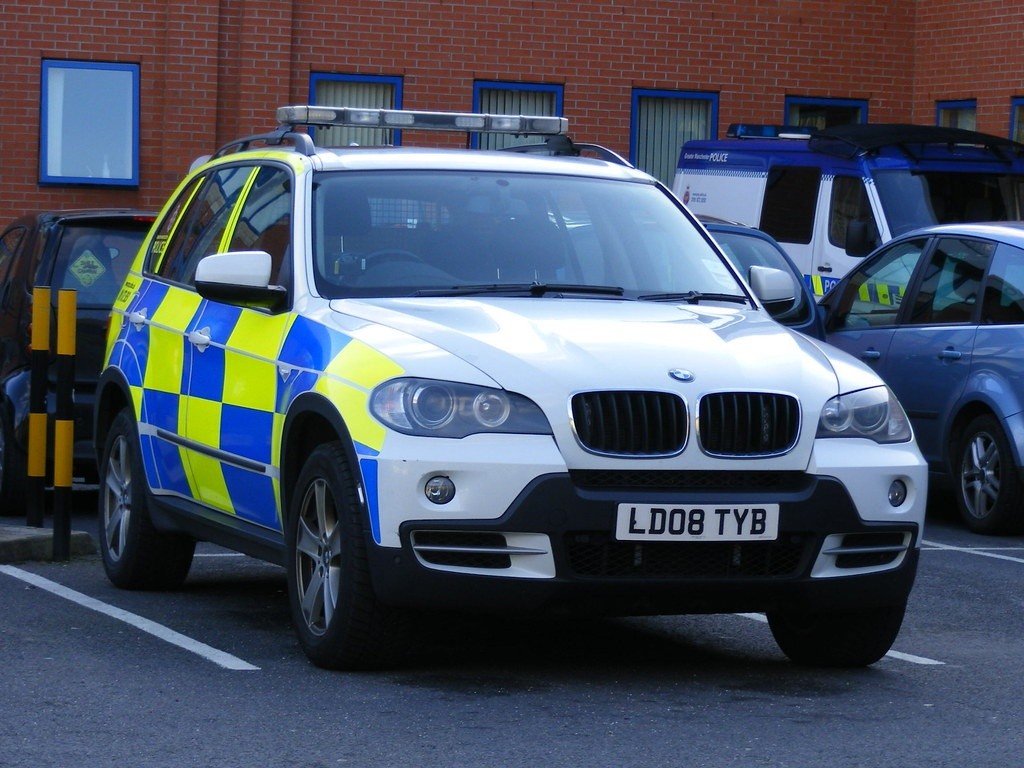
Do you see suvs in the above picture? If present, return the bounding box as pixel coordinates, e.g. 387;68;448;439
94;105;929;669
0;208;238;516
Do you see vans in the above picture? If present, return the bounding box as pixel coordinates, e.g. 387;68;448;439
671;122;1023;328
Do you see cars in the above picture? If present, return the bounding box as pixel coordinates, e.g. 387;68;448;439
816;219;1024;535
562;212;825;341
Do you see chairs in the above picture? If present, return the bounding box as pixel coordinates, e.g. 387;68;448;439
324;188;372;275
490;217;565;282
938;256;989;322
64;241;119;303
